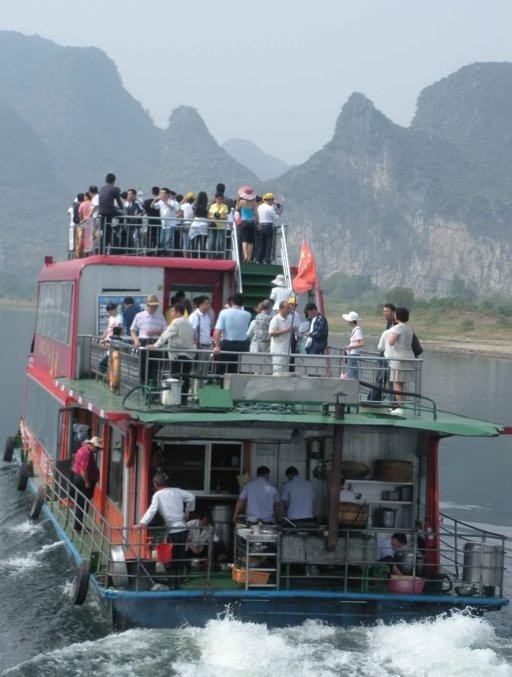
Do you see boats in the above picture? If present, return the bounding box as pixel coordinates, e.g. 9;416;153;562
3;208;511;628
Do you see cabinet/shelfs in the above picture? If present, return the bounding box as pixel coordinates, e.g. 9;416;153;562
340;477;419;569
232;528;278;591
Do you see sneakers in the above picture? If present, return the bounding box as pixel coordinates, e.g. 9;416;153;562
391;407;407;416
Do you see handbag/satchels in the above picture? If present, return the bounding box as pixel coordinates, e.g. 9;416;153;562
412;330;424;359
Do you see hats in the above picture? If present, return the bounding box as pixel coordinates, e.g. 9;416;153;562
146;294;159;306
271;274;288;286
342;311;362;323
238;186;257;200
85;436;104;450
288;298;298;305
262;193;274;202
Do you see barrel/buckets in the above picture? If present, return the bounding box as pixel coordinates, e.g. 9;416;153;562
161;378;182;405
380;509;397;528
154;534;173;561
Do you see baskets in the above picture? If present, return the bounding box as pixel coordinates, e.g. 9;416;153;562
231;565;271;587
